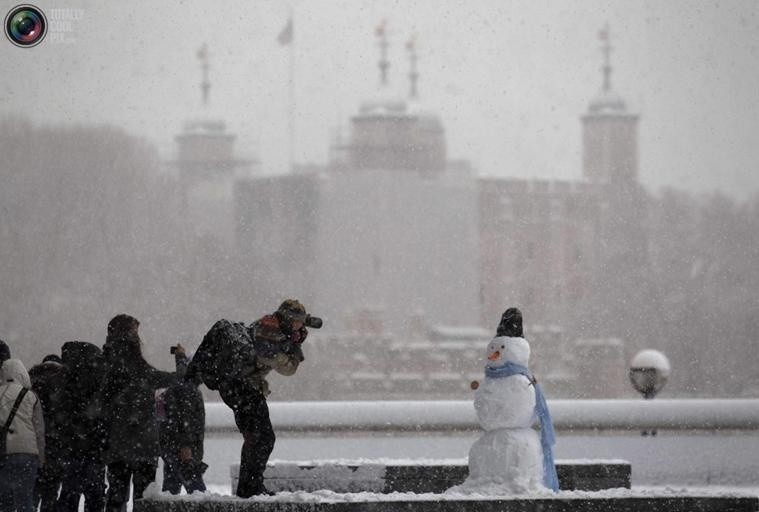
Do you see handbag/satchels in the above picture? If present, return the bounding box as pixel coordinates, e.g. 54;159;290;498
0;426;9;468
92;416;109;452
188;318;253;390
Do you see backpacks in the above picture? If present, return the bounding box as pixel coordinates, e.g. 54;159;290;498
155;390;170;457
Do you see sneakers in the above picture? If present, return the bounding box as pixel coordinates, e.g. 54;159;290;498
236;482;273;498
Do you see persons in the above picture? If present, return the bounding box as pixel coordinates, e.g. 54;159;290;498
58;335;106;512
220;298;308;500
98;312;197;512
1;356;45;512
1;338;11;368
28;354;76;511
156;381;206;494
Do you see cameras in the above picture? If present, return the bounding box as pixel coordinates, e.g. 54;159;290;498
170;346;178;354
304;314;323;329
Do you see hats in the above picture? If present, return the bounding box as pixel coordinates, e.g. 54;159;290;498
280;299;307;323
61;342;101;367
42;360;62;377
